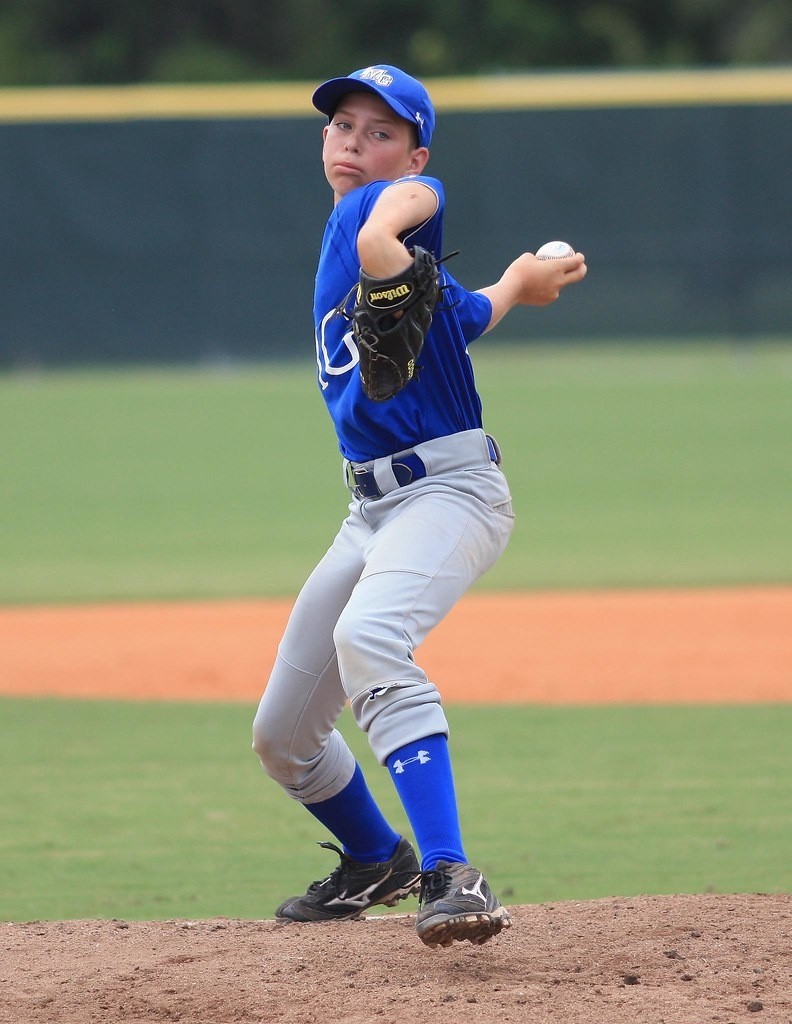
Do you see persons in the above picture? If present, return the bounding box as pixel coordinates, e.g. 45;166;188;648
252;64;587;944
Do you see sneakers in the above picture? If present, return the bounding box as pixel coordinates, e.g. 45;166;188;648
274;833;422;923
394;860;513;948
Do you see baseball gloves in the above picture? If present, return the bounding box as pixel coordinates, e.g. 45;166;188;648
347;243;443;402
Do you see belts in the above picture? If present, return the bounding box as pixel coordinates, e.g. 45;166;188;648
353;432;498;499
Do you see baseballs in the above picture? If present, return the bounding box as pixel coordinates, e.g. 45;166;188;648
534;240;575;261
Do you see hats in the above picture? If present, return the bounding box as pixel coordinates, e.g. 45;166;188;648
312;65;436;150
